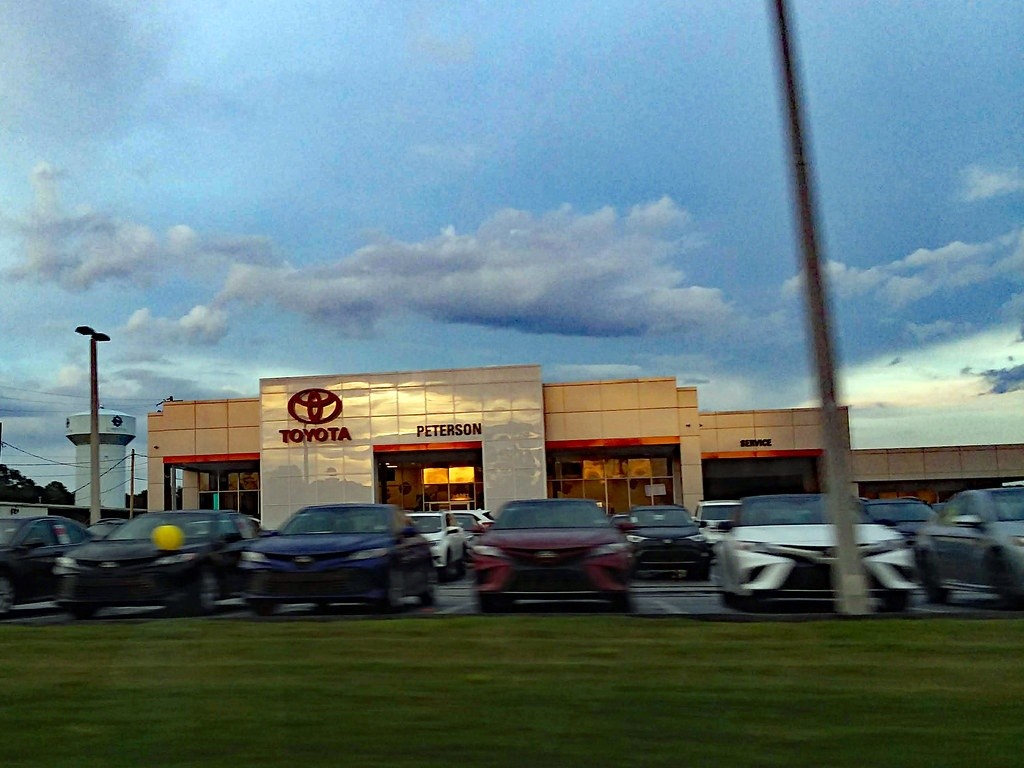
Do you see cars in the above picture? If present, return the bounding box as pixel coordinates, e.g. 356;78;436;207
404;484;1024;616
234;502;436;616
1;508;258;622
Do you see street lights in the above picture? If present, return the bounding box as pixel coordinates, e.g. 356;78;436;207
74;324;112;528
